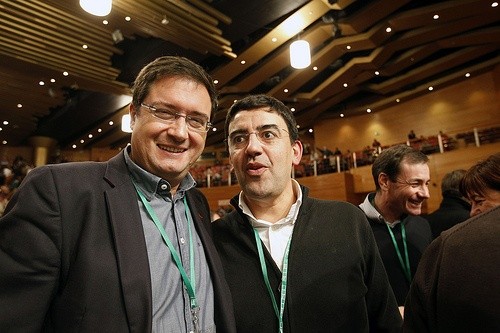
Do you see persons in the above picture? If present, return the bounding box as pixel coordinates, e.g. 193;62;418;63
460;152;500;219
0;55;236;333
191;159;242;189
209;94;409;333
410;124;499;156
0;157;36;216
431;169;471;235
294;138;383;178
401;204;500;333
356;147;439;310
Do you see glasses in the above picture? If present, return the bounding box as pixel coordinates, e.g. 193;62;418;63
140;102;213;132
224;126;291;146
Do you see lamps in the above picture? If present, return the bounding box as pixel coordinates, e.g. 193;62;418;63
290;33;312;69
